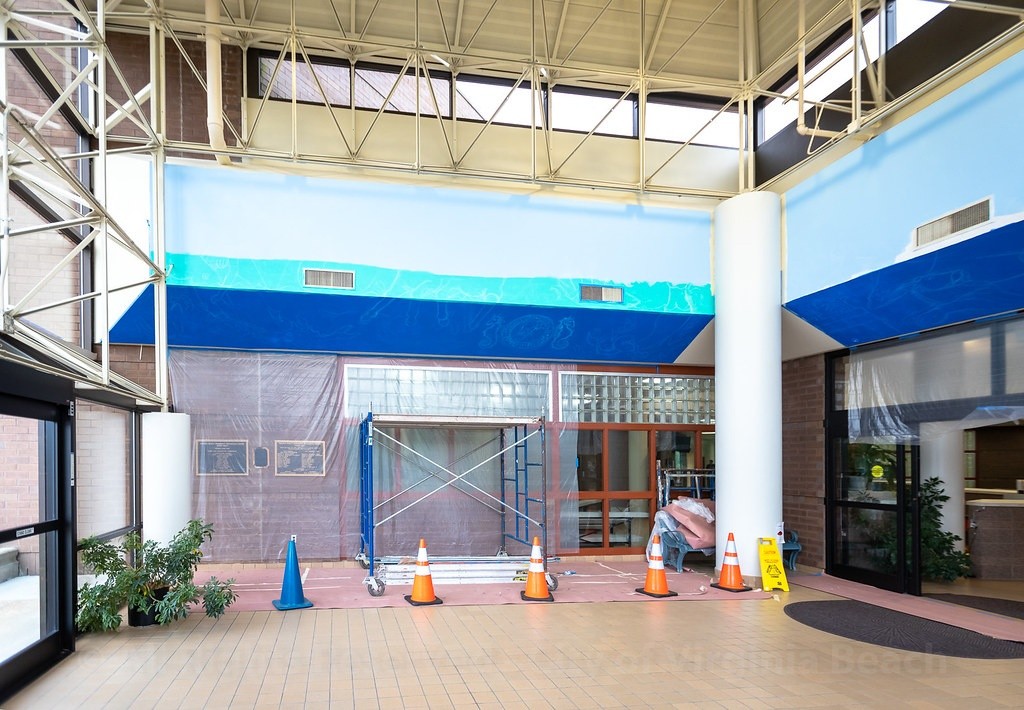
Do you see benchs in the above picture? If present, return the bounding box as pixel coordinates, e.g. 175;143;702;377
655;512;801;574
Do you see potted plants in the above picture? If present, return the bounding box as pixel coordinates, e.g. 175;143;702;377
846;443;971;584
76;518;239;633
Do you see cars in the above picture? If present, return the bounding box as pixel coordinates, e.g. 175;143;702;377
842;473;914;532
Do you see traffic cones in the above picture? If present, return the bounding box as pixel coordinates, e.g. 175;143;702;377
520;536;554;602
634;533;679;598
272;540;313;610
709;533;753;592
403;537;443;606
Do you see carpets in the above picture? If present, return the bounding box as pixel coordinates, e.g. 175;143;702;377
923;593;1024;619
